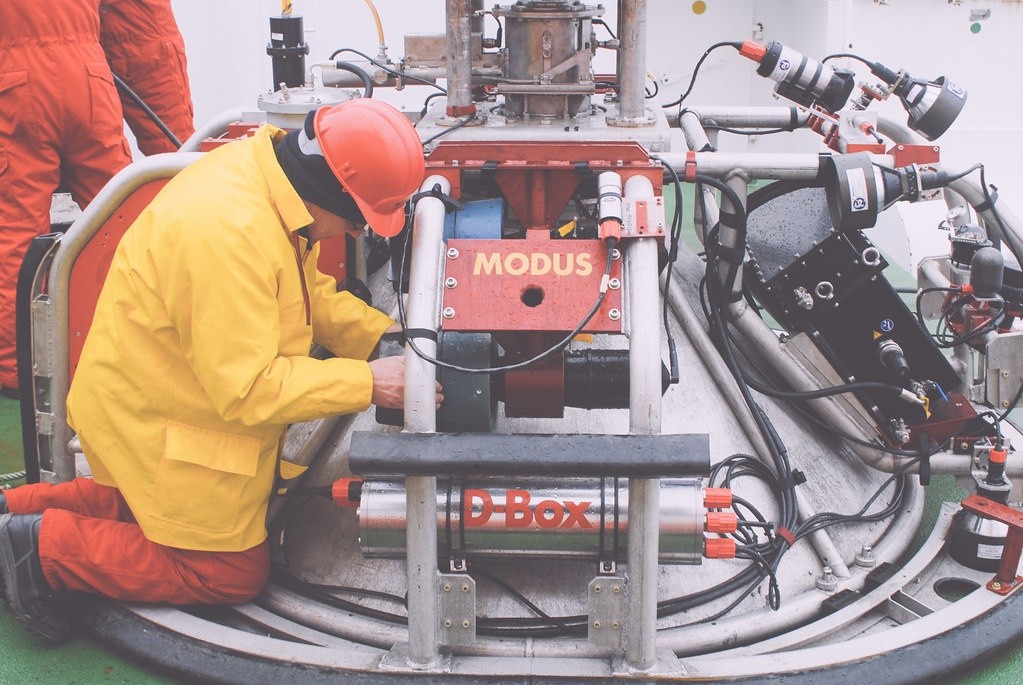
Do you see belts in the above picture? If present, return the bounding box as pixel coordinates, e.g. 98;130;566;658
0;513;70;642
0;493;8;514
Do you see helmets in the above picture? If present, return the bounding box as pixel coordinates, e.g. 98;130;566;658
311;98;425;238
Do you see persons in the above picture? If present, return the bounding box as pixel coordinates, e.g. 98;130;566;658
98;0;195;155
0;0;135;401
0;97;445;645
